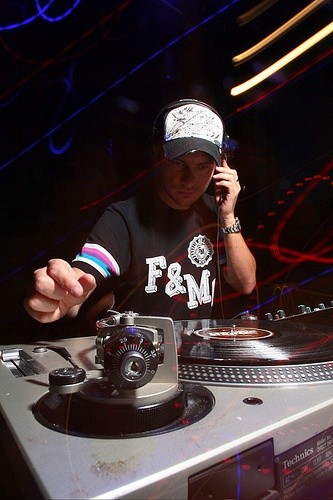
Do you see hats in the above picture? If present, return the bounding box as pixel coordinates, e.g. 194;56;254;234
148;99;224;169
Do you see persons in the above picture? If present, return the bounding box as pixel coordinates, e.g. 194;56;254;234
23;95;259;340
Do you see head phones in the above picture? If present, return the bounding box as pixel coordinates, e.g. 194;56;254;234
148;98;231;165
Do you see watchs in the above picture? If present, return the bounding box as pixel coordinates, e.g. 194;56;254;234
219;217;242;234
